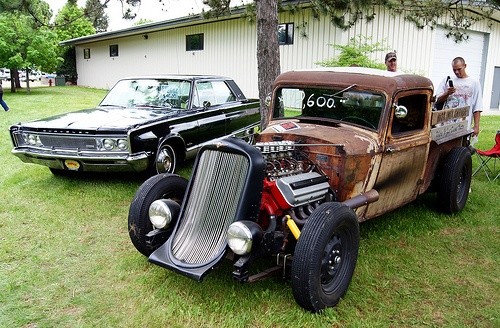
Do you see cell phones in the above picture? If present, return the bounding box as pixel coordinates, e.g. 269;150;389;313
449;80;453;89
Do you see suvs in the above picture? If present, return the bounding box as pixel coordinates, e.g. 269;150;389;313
128;68;478;313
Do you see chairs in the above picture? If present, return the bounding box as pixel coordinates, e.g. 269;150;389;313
470;129;500;186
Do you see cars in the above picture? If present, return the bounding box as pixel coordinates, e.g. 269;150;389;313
8;75;261;181
1;68;49;81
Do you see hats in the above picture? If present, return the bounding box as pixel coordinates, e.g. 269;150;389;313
385;52;397;61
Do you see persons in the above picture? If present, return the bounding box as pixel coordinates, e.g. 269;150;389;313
376;52;404;107
0;80;9;112
433;56;483;146
